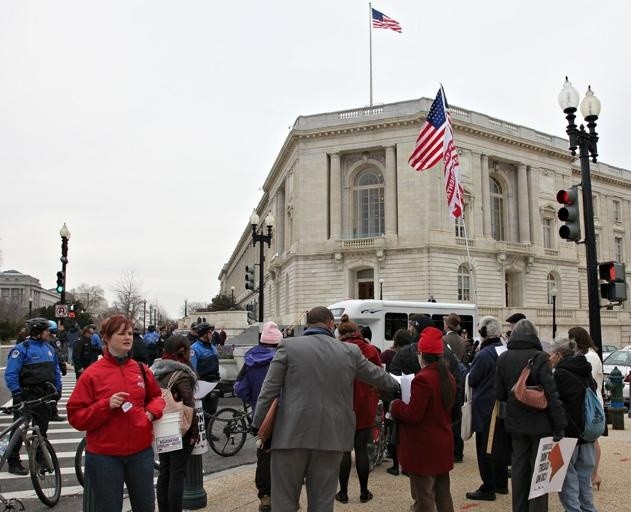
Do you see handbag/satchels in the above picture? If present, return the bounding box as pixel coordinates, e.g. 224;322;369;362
511;358;547;409
461;400;473;441
153;388;193;436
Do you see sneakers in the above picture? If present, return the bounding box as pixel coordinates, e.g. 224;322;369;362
259;495;271;512
360;492;372;502
9;464;28;475
387;467;399;475
336;491;347;503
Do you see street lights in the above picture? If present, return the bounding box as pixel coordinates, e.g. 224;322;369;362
28;296;34;319
551;284;558;340
379;278;385;299
231;285;236;309
184;298;188;317
58;221;70;336
249;208;275;323
149;302;162;328
557;73;604;399
143;297;147;336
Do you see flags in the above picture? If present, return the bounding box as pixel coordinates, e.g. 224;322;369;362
442;113;466;220
372;8;402;33
407;86;448;172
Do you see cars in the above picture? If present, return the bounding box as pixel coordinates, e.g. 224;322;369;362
172;328;191;338
540;341;631;405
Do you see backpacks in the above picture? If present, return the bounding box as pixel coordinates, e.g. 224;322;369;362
564;369;606;441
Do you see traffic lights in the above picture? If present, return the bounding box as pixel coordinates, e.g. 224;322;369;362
244;263;256;291
247;297;257;325
597;258;628;303
56;271;64;293
557;185;581;244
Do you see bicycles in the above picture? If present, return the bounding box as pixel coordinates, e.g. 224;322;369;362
73;434;163;490
1;382;63;511
364;404;393;472
207;388;255;458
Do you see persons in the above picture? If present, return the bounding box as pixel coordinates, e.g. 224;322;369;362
466;313;604;512
236;304;475;511
5;316;227;510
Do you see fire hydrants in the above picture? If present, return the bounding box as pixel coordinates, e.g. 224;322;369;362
604;365;628;429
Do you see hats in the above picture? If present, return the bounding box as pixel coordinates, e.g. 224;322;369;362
479;313;537;338
260;321;283;344
410;313;462;353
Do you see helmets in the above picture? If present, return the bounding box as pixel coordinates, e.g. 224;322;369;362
193;323;214;334
25;318;50;330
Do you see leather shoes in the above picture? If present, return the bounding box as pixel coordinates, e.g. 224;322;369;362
466;487;508;500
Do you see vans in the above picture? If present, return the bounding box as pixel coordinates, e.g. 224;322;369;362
320;293;480;354
